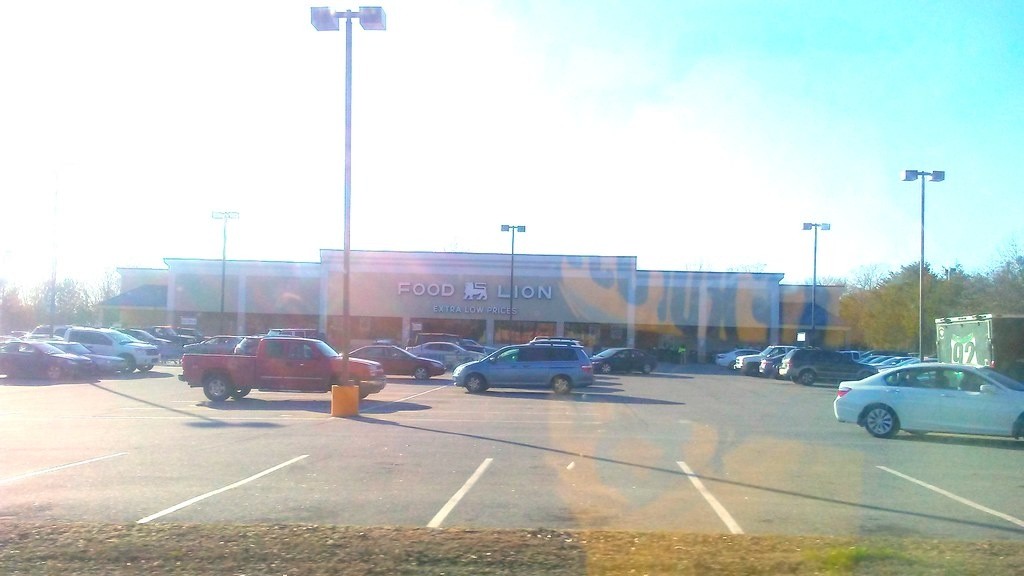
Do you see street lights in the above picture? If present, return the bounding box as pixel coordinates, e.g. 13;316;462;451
212;211;239;335
502;225;526;346
901;169;945;363
311;5;386;385
803;223;831;347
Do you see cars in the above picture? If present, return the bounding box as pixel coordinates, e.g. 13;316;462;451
716;349;762;370
0;340;92;379
405;342;487;371
840;350;939;373
47;341;124;377
461;339;497;355
0;331;64;343
833;365;1024;440
758;354;792;380
590;348;657;374
349;346;446;380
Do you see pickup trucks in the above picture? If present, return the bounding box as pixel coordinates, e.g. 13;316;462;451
150;325;197;347
174;328;210;343
178;337;386;402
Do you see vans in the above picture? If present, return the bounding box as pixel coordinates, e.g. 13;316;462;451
267;329;316;339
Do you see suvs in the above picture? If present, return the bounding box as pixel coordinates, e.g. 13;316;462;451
779;347;878;385
111;329;171;363
65;328;160;372
452;336;594;395
734;346;797;376
407;333;486;354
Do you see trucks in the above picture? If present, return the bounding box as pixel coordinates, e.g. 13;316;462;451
933;313;1024;385
